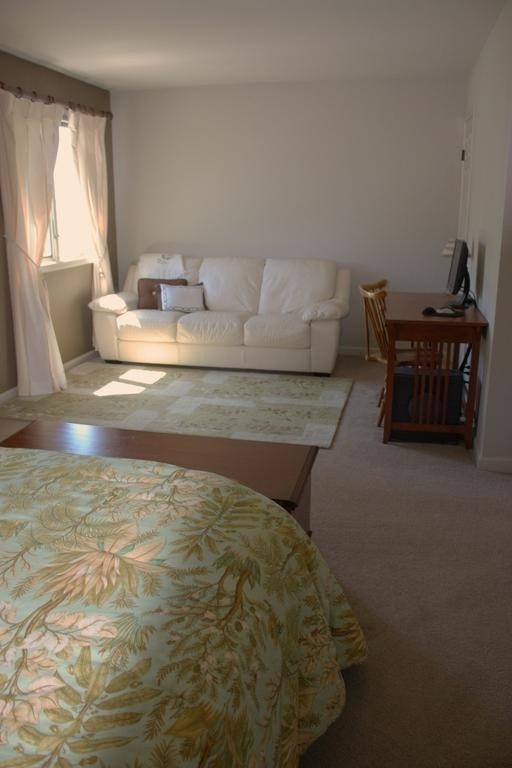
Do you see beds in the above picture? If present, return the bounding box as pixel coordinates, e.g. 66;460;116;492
0;448;371;766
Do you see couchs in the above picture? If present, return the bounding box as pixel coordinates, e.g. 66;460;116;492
87;253;351;377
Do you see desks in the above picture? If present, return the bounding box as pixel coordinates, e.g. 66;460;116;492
1;416;317;540
382;290;488;451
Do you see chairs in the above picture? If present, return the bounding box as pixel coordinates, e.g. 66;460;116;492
361;279;439;428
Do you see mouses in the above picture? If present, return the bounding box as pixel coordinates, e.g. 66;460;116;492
436;306;456;314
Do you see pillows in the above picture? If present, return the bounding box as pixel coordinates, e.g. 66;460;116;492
158;282;207;314
137;277;187;310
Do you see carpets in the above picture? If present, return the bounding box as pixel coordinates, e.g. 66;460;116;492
0;361;354;449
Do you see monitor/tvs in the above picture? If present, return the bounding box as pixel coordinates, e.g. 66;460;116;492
445;239;471;308
